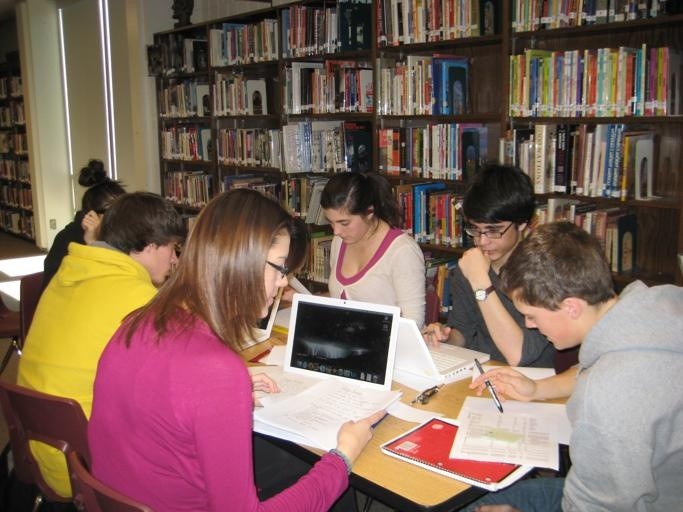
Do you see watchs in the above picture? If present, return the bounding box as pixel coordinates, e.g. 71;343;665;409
473;285;496;302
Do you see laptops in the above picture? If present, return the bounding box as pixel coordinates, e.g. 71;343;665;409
235;285;283;351
394;318;491;381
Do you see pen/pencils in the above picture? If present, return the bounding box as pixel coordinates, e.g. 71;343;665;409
423;323;453;335
474;358;504;414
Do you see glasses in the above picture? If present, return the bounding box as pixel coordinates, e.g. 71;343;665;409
464;222;514;239
266;260;290;278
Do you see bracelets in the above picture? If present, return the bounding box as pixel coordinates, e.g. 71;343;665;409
328;449;352;476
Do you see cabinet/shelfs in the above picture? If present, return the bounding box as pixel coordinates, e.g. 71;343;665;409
152;0;682;325
1;1;38;243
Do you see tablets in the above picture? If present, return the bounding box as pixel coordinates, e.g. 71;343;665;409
283;293;401;391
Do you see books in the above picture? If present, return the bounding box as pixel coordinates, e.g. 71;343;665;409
0;0;37;241
154;1;681;320
380;417;536;492
471;364;557;386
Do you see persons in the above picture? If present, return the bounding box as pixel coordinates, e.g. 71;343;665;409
317;171;427;330
42;156;127;287
418;158;557;368
463;220;682;511
13;189;188;501
86;186;388;511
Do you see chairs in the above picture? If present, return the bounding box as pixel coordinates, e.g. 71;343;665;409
1;271;440;512
